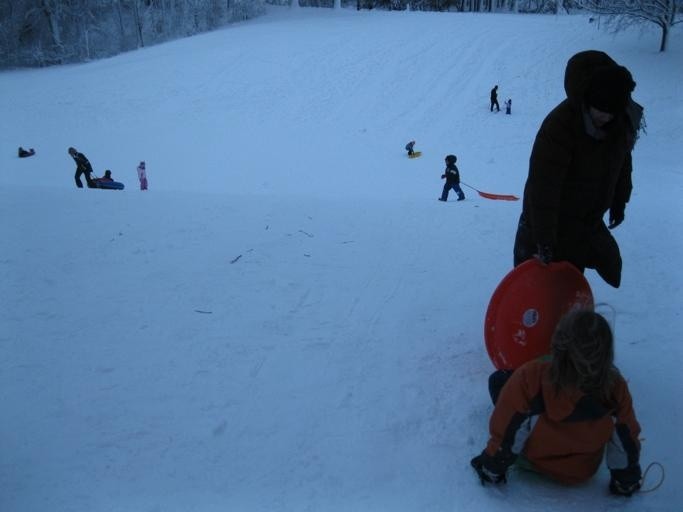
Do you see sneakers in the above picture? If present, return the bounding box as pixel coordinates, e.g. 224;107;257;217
438;196;465;201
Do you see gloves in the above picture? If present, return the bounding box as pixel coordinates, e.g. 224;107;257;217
610;478;641;498
471;453;506;487
608;205;625;229
537;242;553;264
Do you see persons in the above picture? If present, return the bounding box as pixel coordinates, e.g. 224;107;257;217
18;145;35;157
485;50;645;404
467;309;645;497
405;141;417;156
138;160;148;191
491;84;502;112
64;146;97;189
504;99;511;114
438;155;466;201
91;170;115;182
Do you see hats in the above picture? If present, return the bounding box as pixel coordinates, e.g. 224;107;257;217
445;155;456;163
